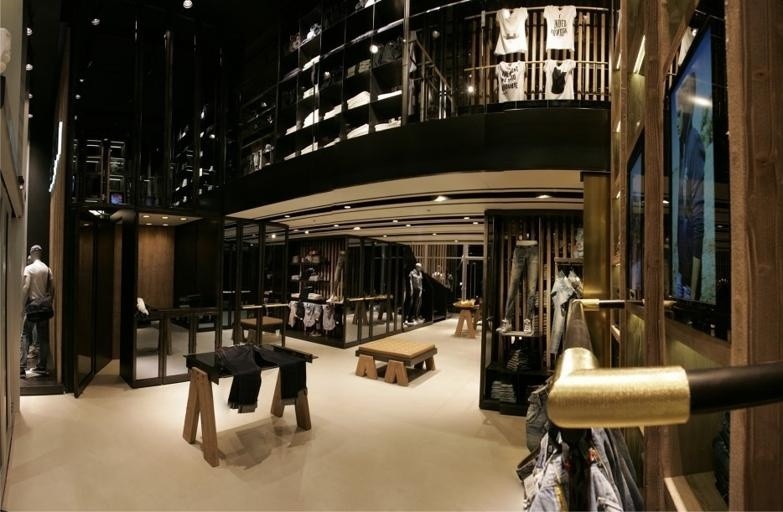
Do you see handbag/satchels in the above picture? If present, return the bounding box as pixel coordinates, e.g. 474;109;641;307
25;296;53;322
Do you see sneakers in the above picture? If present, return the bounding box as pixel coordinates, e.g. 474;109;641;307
20;370;25;378
32;368;46;374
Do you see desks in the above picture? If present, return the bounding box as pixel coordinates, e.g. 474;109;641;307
182;343;317;467
453;298;480;338
345;294;394;325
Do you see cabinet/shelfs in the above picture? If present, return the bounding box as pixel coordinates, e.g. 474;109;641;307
226;1;409;180
287;260;329;303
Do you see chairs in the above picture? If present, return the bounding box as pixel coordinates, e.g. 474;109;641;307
230;304;286;347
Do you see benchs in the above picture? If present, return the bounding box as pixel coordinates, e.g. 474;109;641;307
354;335;437;385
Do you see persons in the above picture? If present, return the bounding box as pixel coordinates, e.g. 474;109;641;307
408;263;424;323
431;265;445;286
675;73;705;302
19;244;54;378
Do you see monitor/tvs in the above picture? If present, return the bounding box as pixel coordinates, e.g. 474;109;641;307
663;21;732;346
626;124;644;306
